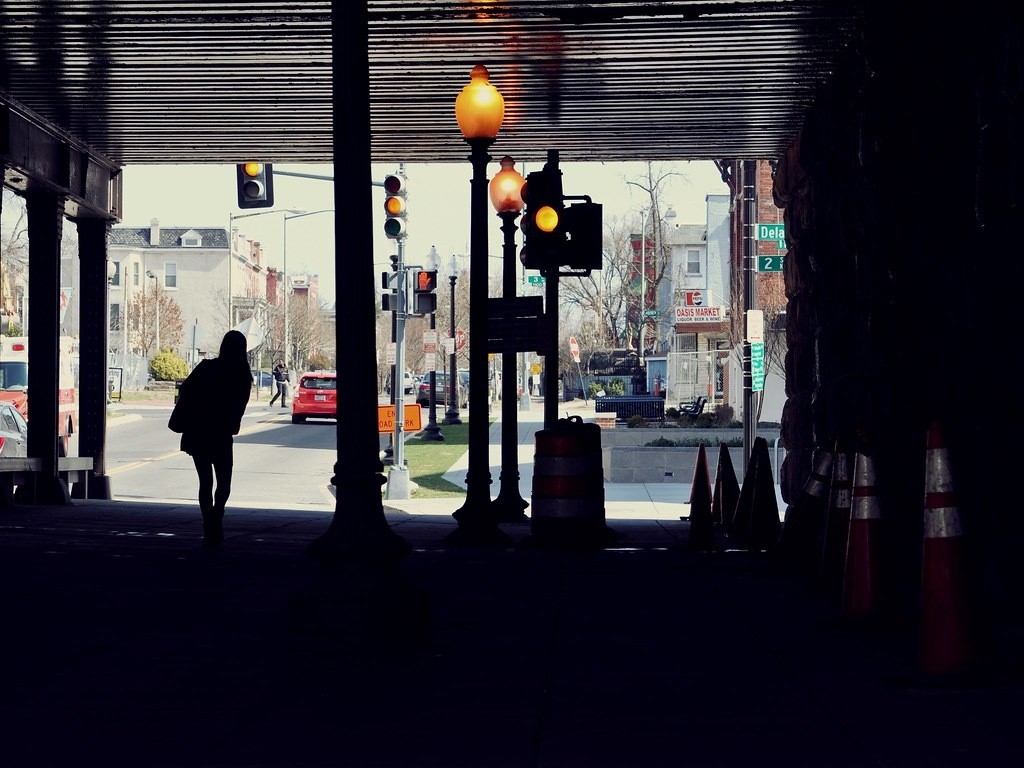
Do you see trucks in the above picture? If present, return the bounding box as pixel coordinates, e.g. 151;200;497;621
0;336;77;457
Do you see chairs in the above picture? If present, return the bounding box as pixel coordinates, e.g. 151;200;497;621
679;396;707;419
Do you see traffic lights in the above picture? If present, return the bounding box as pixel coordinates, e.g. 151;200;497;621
381;294;398;311
241;163;266;202
521;171;564;270
414;293;439;314
566;203;604;270
384;173;408;239
414;269;438;292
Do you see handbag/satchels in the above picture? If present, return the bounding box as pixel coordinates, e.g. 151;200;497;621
167;398;184;433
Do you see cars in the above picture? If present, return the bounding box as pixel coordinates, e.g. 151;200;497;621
291;371;337;424
387;370;524;407
251;370;274;386
0;402;29;458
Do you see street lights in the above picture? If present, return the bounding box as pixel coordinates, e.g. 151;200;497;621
453;64;531;534
146;270;160;350
228;208;307;331
637;209;678;365
441;253;462;425
424;244;445;441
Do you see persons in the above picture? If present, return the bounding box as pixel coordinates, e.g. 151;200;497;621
269;359;291;408
179;331;253;551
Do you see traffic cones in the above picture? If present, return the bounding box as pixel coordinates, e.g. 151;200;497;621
771;427;877;626
687;437;783;551
917;418;981;679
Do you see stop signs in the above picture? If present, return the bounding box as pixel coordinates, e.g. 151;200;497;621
570;337;579;357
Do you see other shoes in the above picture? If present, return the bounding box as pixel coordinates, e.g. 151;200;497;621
281;405;287;407
270;402;273;407
202;531;225;547
